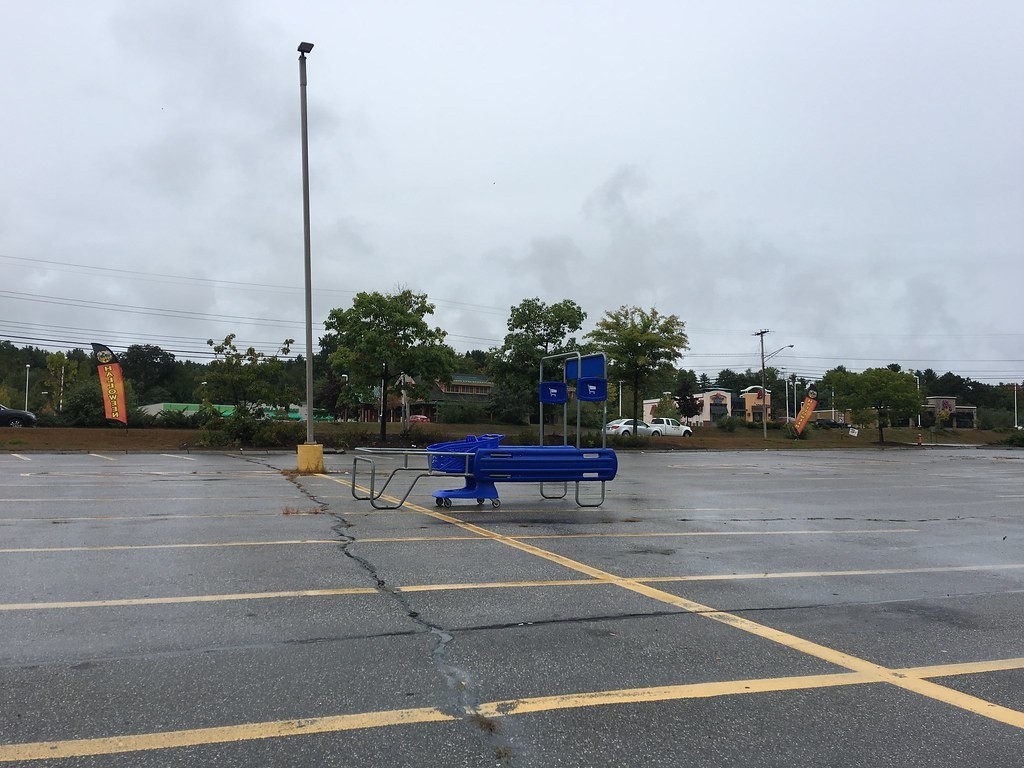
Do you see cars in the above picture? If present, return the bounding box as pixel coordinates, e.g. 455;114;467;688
650;418;693;438
0;404;37;428
602;418;650;436
816;418;843;428
776;417;796;427
405;415;431;424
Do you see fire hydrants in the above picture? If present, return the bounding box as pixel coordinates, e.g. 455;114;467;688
916;434;924;446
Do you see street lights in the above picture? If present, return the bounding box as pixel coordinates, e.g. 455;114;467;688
297;40;324;472
24;362;31;411
795;382;802;420
762;344;794;439
913;375;922;428
619;379;624;417
